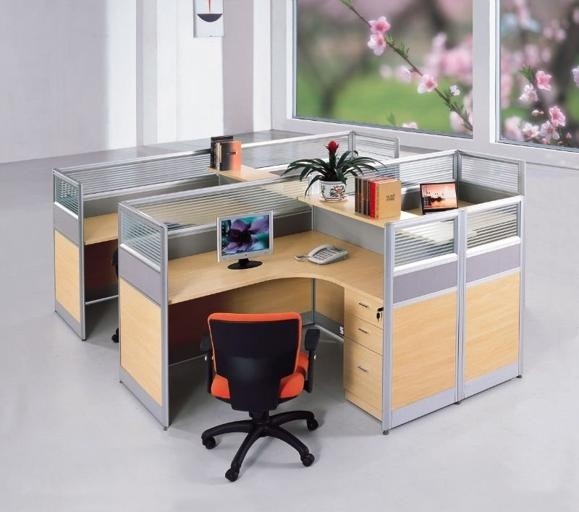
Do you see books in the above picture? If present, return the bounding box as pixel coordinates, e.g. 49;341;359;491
355;175;403;219
211;136;241;171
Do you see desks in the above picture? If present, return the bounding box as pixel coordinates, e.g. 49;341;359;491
53;129;527;434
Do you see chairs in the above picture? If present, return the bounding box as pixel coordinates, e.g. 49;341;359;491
199;312;322;483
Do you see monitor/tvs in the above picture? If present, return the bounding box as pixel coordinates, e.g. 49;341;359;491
217;209;273;269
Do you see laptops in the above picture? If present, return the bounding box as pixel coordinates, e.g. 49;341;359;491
420;182;458;215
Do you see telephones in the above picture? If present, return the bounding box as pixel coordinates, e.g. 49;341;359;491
294;243;349;265
